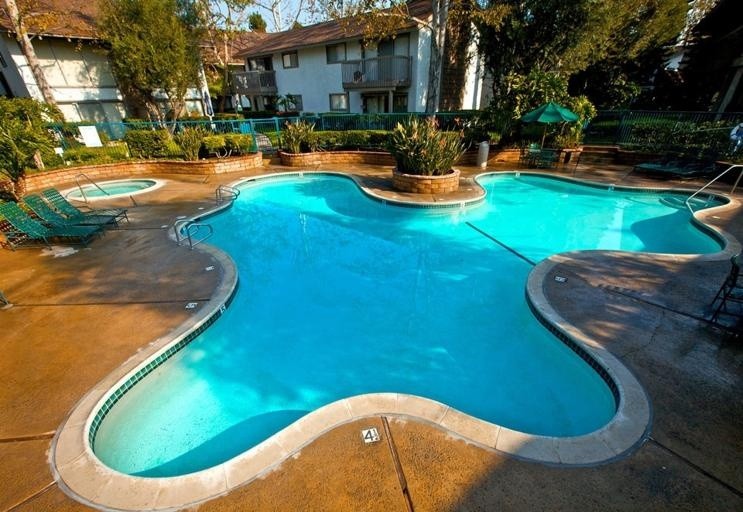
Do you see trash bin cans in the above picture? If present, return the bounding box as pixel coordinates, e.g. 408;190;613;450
475;140;490;168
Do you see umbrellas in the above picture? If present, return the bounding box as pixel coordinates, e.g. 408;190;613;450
521;101;579;150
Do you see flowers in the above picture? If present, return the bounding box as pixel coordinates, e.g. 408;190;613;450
384;112;469;175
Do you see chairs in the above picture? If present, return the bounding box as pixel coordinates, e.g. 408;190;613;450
1;188;131;251
517;143;554;170
